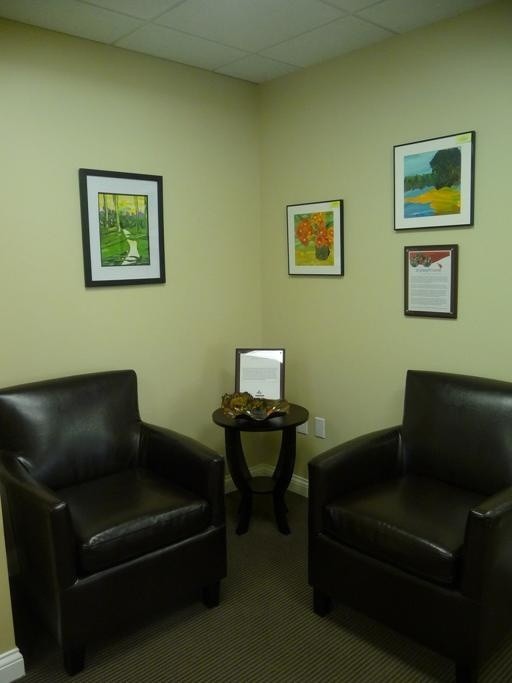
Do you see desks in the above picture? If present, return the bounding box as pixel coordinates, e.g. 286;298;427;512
211;403;308;535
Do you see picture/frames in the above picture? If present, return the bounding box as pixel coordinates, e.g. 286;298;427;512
235;347;284;402
392;129;475;231
403;242;457;319
78;166;166;287
285;199;345;277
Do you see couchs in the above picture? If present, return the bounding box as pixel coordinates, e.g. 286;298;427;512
305;366;511;682
1;368;230;677
